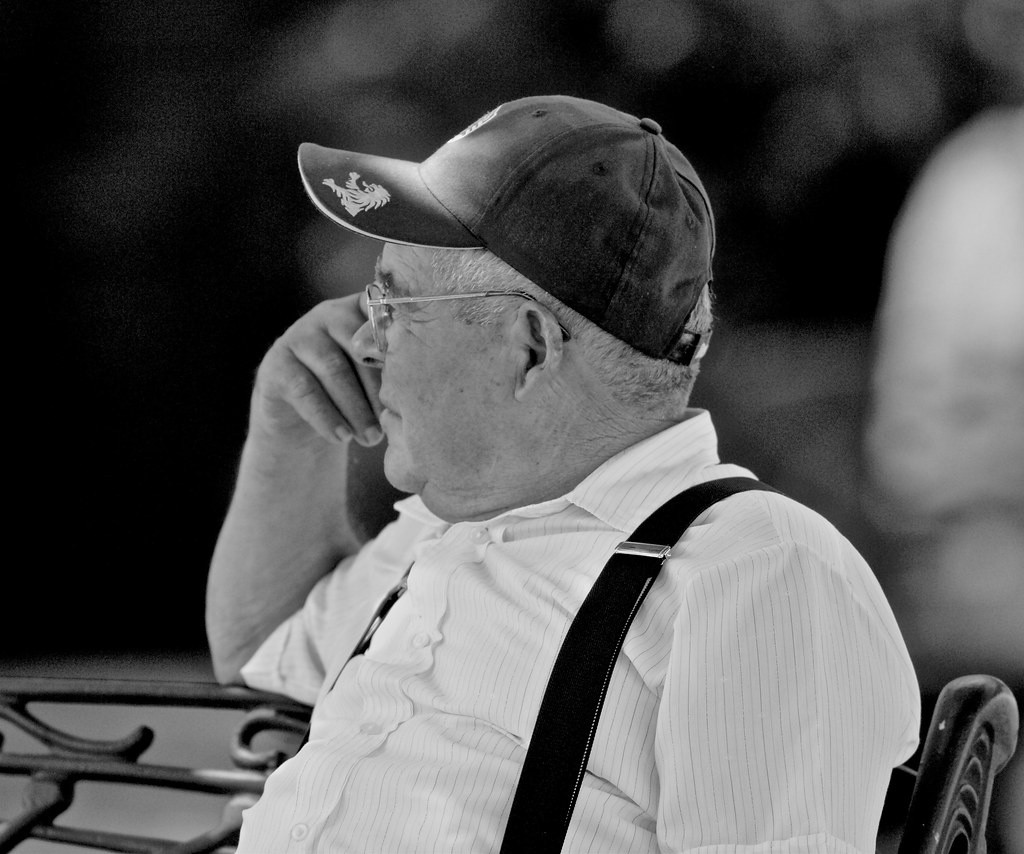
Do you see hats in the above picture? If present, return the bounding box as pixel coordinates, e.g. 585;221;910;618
297;94;717;366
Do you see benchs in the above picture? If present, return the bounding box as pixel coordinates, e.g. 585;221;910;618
0;673;1020;854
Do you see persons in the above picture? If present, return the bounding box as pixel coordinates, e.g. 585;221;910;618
203;98;922;854
864;0;1024;853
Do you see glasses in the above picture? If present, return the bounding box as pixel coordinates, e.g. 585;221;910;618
365;283;572;353
